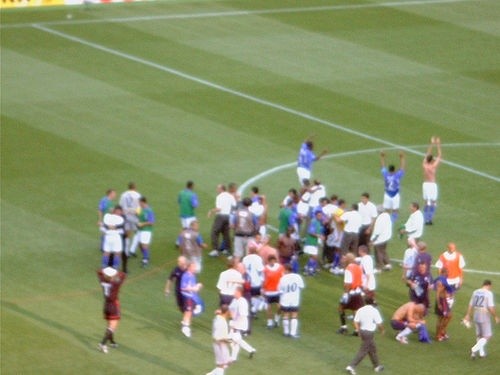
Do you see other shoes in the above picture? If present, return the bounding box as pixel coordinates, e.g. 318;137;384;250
335;267;344;273
443;334;448;339
283;333;289;336
352;331;359;336
275;320;279;328
222;250;229;255
425;223;429;225
374;365;384;372
346;366;357;375
129;251;138;258
231;359;236;363
140;257;148;267
304;270;310;275
208;250;219;256
310;271;315;276
291;334;300;339
267;319;272;329
180;321;191;337
319;260;334;269
227;255;233;260
335;326;347;334
98;343;107;354
436;338;443;342
383;264;392;270
480;353;487;358
248;349;256;359
329;268;339;274
395;335;408;344
109;344;119;347
471;348;476;360
373;269;381;274
430;221;432;225
